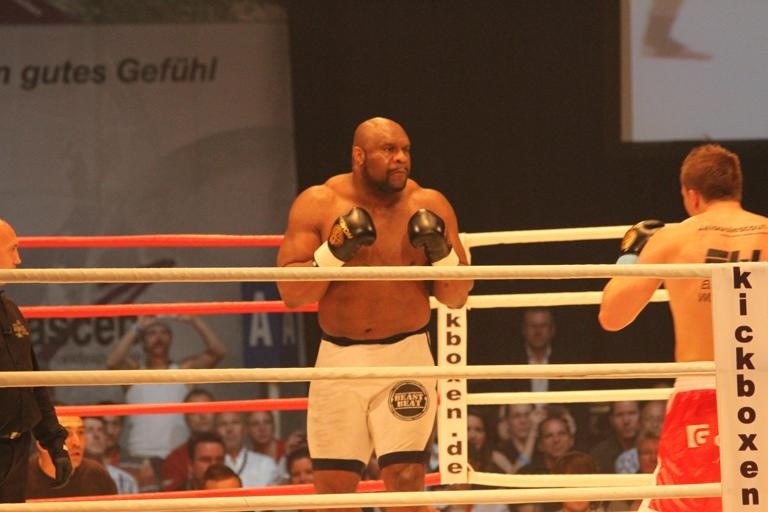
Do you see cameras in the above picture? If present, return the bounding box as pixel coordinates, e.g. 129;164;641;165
545;405;562;422
153;315;177;326
300;431;307;445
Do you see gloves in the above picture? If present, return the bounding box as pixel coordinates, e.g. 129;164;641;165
314;207;376;267
408;210;458;266
49;441;72;488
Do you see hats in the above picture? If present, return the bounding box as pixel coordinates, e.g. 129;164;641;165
615;221;664;263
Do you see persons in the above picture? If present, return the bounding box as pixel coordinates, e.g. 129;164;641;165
0;218;73;502
274;115;477;511
22;308;673;511
599;142;768;511
